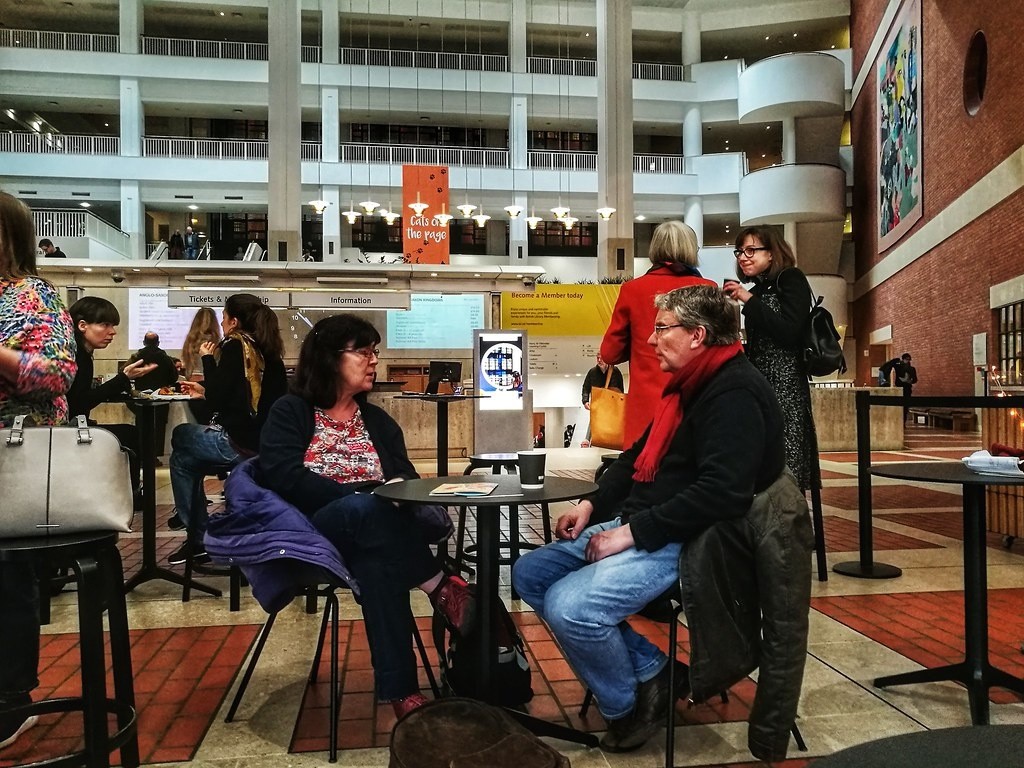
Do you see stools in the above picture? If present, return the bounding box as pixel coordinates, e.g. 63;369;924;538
0;530;141;768
183;463;249;611
456;452;552;601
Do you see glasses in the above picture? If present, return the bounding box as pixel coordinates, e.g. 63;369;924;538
734;246;768;259
656;323;682;338
43;248;49;251
340;346;380;357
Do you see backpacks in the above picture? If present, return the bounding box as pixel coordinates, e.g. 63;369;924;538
777;267;847;381
387;698;572;768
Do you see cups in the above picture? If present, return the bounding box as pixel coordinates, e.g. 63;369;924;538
517;451;548;489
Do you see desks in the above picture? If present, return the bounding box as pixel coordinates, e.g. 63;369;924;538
868;462;1024;726
393;396;491;574
102;393;222;611
374;474;599;748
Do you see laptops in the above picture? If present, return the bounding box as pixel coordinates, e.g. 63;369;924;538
402;361;461;394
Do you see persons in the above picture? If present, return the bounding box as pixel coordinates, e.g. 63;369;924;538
879;353;917;429
39;239;66;258
257;314;474;720
723;223;822;491
68;295;286;564
600;221;718;451
162;226;200;259
583;352;624;442
511;285;785;753
0;191;78;746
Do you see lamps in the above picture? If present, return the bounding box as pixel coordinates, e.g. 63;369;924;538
309;0;617;230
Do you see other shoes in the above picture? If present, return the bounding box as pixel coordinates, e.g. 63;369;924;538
205;499;213;506
167;507;187;532
168;540;208;565
602;658;691;755
0;693;41;749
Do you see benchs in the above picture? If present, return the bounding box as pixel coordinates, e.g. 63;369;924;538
908;407;975;432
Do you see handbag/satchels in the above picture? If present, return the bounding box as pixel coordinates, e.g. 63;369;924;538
447;583;534;702
0;414;135;540
589;362;627;452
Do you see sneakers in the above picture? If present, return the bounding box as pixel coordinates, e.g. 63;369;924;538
392;692;433;721
432;574;477;635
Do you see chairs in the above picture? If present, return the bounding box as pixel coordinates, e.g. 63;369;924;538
202;456;438;762
578;464;815;768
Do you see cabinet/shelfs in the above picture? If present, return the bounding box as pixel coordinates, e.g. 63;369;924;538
387;365;458;395
